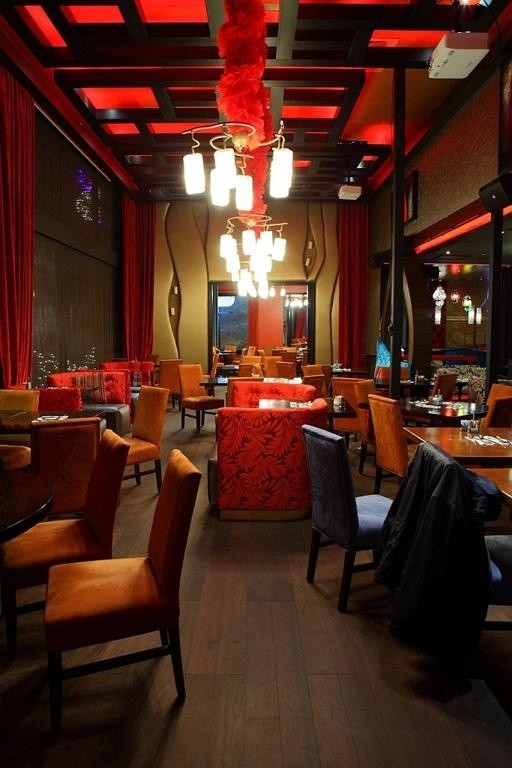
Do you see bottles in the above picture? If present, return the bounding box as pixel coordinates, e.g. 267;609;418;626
434;394;442;405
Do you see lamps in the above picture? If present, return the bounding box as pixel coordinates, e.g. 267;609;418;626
284;293;308;308
432;279;482;326
181;120;294;210
220;214;288;300
